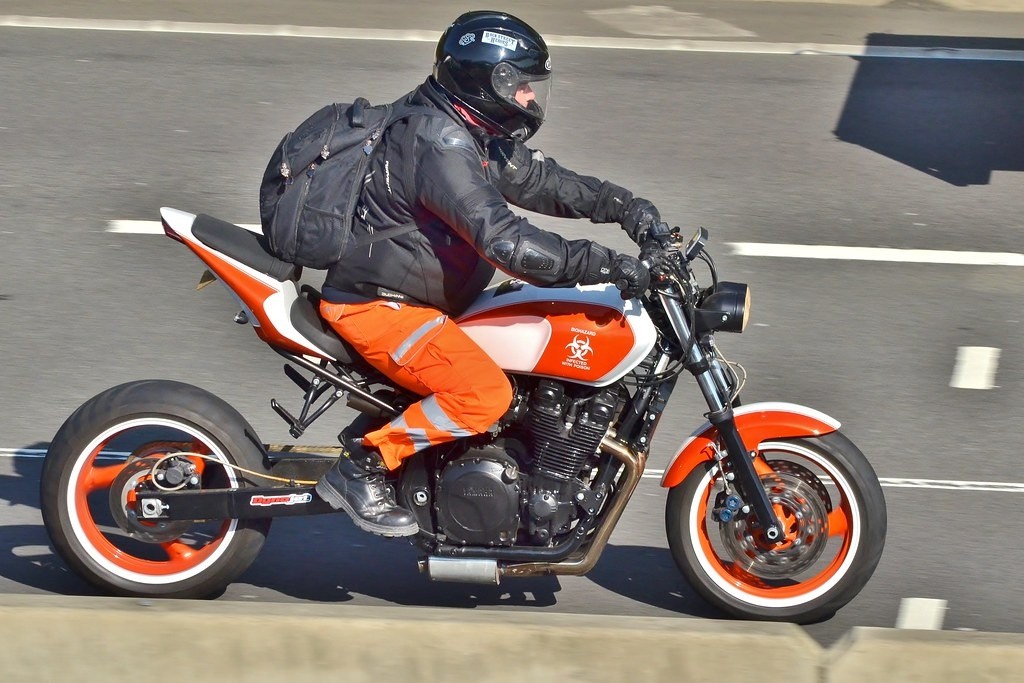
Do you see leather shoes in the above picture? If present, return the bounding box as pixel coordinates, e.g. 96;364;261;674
316;438;419;537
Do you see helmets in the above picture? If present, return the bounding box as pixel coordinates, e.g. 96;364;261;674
432;9;552;143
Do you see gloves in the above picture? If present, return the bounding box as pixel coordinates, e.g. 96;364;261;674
580;241;649;300
590;180;660;243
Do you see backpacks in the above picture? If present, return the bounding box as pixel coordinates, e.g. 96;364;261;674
262;94;463;269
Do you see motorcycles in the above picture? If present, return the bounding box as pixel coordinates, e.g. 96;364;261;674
36;206;891;623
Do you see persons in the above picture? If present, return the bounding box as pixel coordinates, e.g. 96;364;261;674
319;11;661;537
